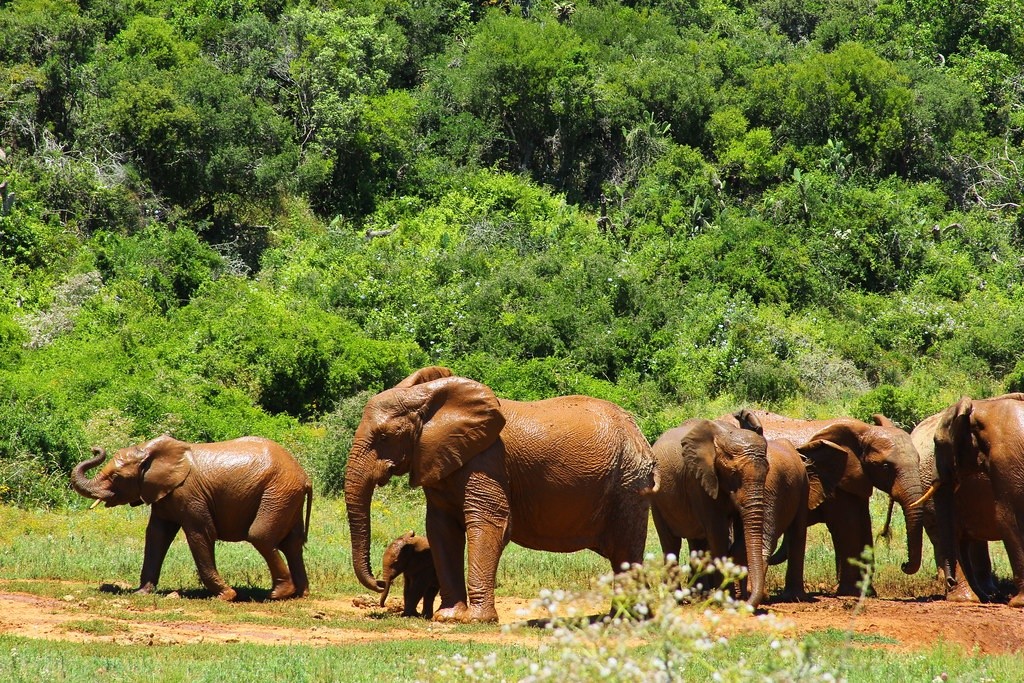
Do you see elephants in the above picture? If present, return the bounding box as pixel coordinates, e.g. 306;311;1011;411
71;434;313;603
649;392;1024;611
342;365;664;626
